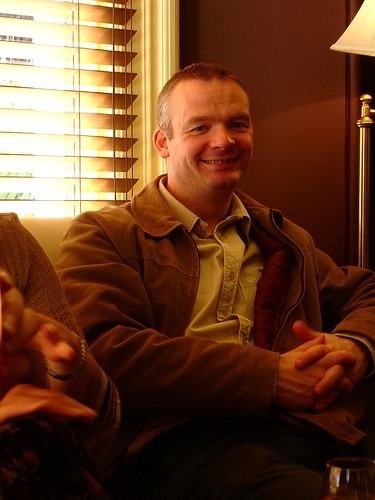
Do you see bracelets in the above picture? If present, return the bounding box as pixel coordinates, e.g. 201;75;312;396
47;340;87;380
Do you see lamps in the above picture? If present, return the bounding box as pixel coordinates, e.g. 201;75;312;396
330;0;375;56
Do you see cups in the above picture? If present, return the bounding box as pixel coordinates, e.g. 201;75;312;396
324;457;375;500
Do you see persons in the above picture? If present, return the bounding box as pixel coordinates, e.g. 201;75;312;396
54;61;375;500
0;213;121;500
